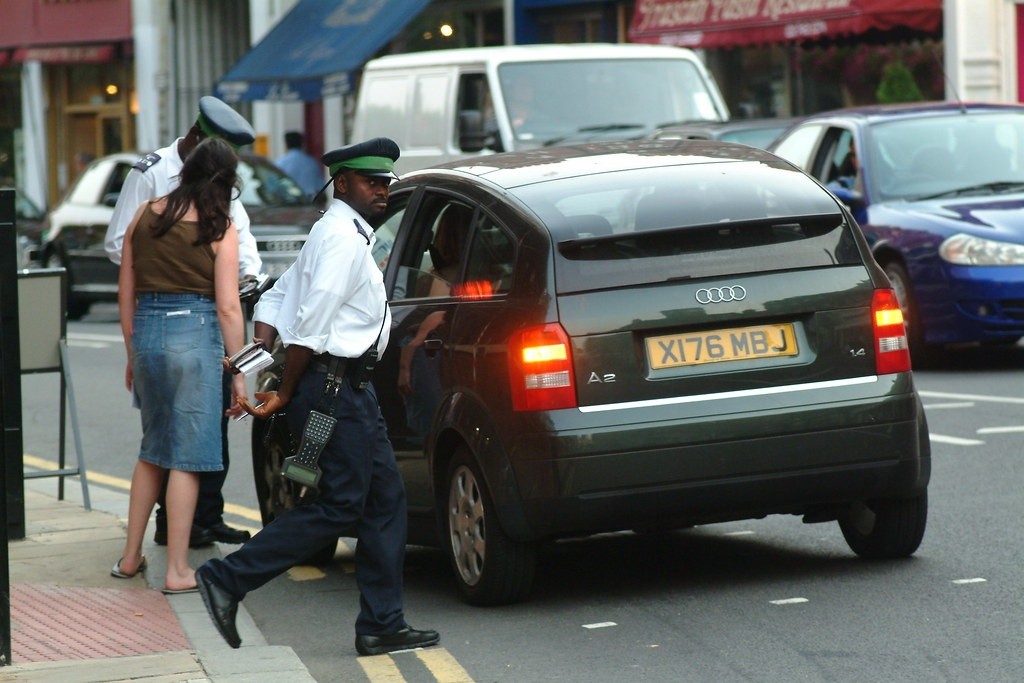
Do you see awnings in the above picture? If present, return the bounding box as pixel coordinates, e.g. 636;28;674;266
211;0;432;105
628;0;943;52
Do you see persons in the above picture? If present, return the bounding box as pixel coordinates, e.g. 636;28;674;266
266;131;324;204
826;136;860;198
415;204;510;298
486;82;531;131
111;137;249;594
194;137;440;657
105;95;263;548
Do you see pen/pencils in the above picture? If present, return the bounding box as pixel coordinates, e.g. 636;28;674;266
235;402;264;424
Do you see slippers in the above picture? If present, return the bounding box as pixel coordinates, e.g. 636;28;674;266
161;572;202;593
111;555;147;578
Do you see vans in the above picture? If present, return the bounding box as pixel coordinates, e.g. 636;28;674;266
351;43;736;180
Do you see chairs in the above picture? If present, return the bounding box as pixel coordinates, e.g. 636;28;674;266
426;199;511;289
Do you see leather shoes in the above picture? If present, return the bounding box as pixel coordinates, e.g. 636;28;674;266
153;517;215;544
355;622;440;656
195;565;242;648
202;521;251;544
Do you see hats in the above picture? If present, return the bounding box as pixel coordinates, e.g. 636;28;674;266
321;137;401;182
198;96;256;154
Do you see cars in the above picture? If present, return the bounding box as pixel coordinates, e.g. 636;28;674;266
38;150;326;322
642;100;1023;357
250;136;933;607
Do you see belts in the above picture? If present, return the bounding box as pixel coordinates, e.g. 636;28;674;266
308;359;353;377
